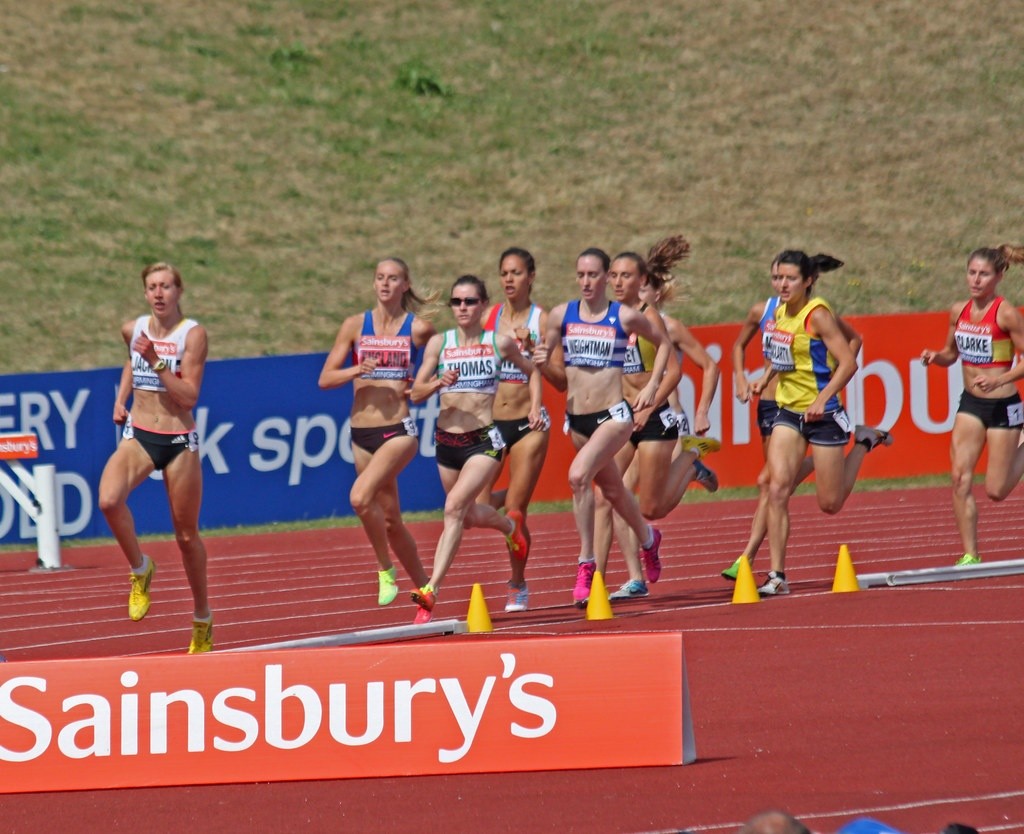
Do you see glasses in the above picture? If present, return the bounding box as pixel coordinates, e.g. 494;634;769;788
448;296;483;307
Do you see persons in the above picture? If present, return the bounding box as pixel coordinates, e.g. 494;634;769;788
920;243;1024;565
99;262;211;652
575;235;721;607
535;247;671;609
477;247;565;611
411;274;546;615
719;252;892;595
317;256;432;624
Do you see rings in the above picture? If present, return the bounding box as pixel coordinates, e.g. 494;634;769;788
522;327;524;329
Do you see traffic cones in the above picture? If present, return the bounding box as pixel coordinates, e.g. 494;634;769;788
465;582;496;633
730;556;762;604
584;570;614;621
829;542;862;593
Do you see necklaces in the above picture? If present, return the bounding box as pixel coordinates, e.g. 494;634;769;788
584;306;594;317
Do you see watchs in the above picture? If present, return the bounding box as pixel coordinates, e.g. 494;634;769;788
153;361;166;370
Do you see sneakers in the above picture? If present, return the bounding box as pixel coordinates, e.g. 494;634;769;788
377;566;399;605
679;434;722;461
573;562;596;605
720;556;753;581
128;555;155;622
693;459;718;494
411;584;438;612
503;511;528;561
504;579;529;612
608;579;649;599
757;571;790;596
640;526;662;583
413;606;434;625
952;552;982;567
188;617;212;655
853;423;893;453
